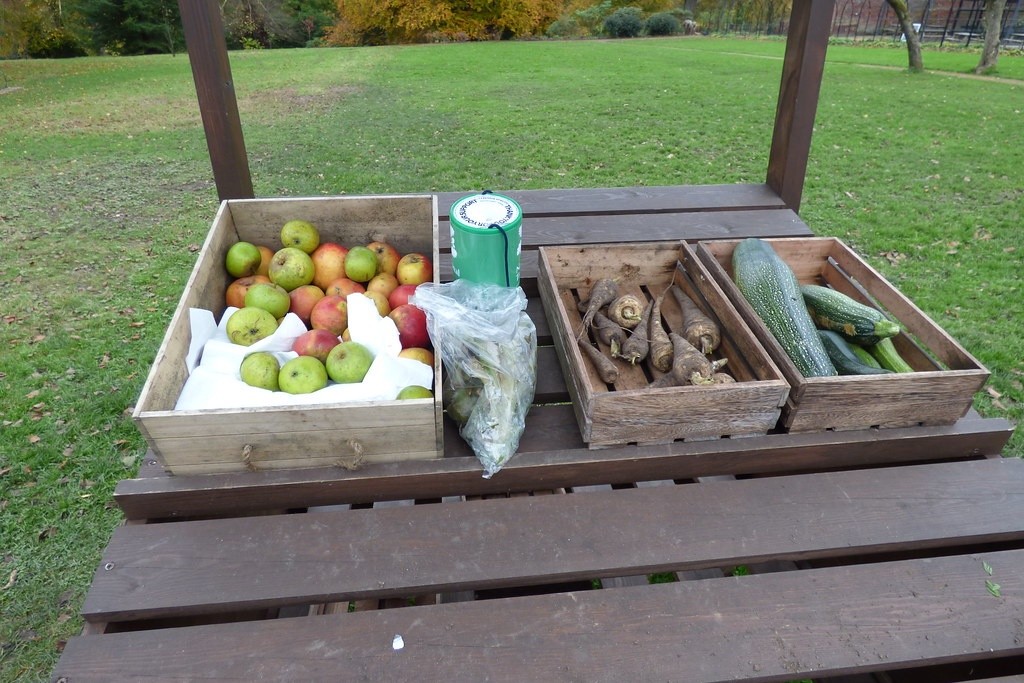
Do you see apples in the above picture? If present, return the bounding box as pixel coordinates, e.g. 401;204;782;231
221;220;437;402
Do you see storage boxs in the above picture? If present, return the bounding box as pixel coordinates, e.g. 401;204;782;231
697;237;991;435
131;194;445;478
537;240;792;452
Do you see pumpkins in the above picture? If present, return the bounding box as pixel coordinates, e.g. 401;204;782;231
732;237;918;378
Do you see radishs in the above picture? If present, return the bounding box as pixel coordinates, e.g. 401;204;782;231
578;277;740;390
